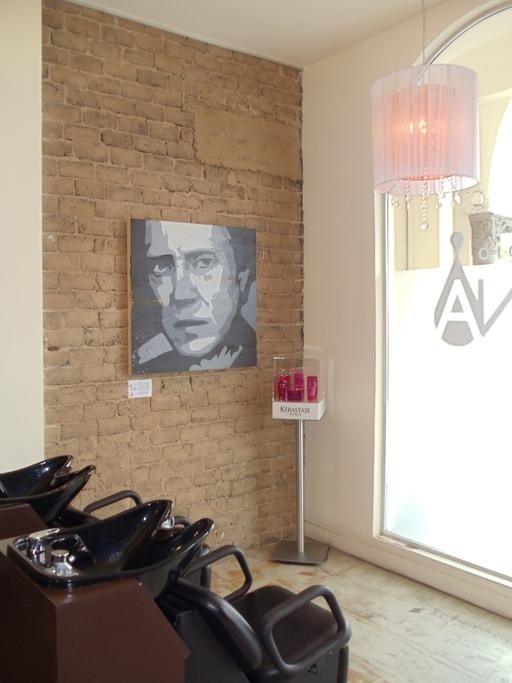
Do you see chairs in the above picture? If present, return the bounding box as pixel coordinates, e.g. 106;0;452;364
165;544;351;682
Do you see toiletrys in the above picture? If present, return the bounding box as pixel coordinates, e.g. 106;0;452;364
276;371;317;401
275;370;317;403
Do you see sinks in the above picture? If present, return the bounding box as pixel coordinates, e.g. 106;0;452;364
10;498;214;586
2;455;95;520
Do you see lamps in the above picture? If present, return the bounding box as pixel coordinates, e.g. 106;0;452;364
370;0;478;232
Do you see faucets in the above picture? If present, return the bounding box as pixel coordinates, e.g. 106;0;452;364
28;524;62;557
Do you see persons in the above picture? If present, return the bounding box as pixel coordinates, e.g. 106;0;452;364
130;217;257;371
